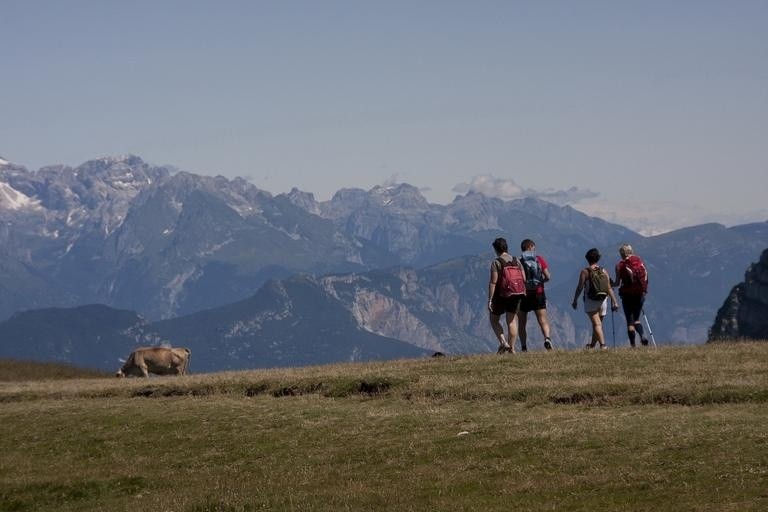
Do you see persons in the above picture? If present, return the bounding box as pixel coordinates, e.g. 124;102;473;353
518;239;552;351
610;244;648;347
572;248;619;350
489;238;526;354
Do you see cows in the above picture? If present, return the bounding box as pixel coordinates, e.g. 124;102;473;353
115;346;192;379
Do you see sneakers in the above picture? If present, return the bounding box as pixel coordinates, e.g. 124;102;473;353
641;336;648;346
585;343;608;350
544;339;553;351
498;342;510;353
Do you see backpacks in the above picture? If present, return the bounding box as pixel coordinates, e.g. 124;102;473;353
496;256;527;301
585;266;608;301
521;255;544;288
621;256;648;295
